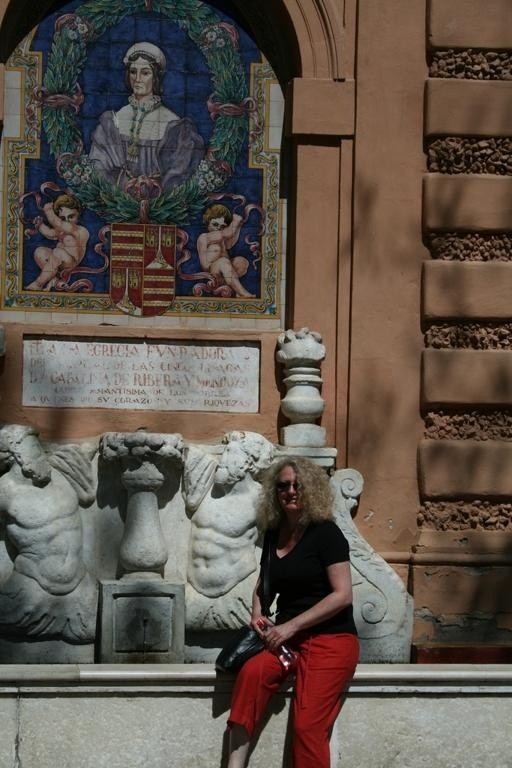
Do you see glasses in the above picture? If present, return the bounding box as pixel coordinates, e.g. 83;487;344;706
278;481;307;492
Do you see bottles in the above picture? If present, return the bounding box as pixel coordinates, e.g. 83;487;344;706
255;617;297;667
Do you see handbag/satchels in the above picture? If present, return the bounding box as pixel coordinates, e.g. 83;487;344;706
215;624;265;673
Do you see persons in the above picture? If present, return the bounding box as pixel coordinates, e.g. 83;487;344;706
217;457;362;767
24;194;89;290
168;431;274;636
87;42;206;199
195;206;257;299
1;421;105;643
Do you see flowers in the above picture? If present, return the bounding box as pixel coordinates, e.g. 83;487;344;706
42;0;249;226
